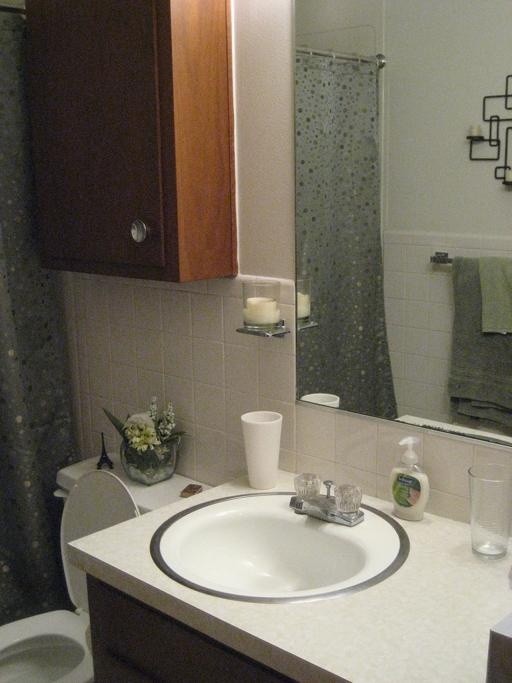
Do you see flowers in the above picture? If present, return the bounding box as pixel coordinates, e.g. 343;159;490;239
101;395;186;484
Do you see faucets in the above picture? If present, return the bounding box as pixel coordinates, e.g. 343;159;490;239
290;496;335;520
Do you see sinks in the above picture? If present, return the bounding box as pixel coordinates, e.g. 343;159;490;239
163;509;367;597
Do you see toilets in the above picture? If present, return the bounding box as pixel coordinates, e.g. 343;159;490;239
0;452;214;683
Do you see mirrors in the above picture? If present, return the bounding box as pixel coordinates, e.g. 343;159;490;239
292;0;512;452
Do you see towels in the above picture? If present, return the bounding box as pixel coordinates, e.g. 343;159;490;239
448;256;512;432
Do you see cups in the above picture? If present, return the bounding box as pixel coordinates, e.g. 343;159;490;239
295;277;312;323
466;459;510;556
242;409;284;488
298;390;341;410
242;280;283;327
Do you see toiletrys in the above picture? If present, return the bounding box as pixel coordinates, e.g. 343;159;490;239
388;435;430;521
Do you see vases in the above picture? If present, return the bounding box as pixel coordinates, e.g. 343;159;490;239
120;439;178;484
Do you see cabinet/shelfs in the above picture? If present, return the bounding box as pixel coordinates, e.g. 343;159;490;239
86;576;294;683
26;0;237;283
